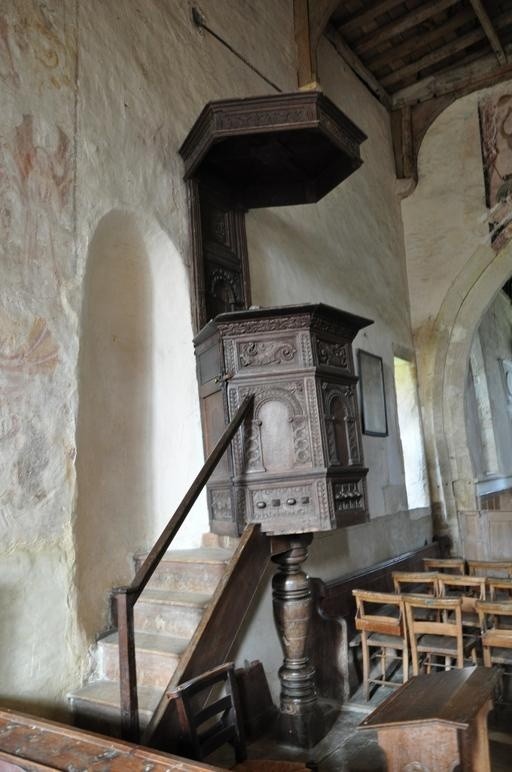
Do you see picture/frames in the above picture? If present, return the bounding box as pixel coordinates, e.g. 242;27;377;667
356;347;390;435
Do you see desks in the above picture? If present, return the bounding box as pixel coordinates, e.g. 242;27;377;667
356;666;504;770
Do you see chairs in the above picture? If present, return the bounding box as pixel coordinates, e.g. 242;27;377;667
167;661;314;770
350;558;512;701
